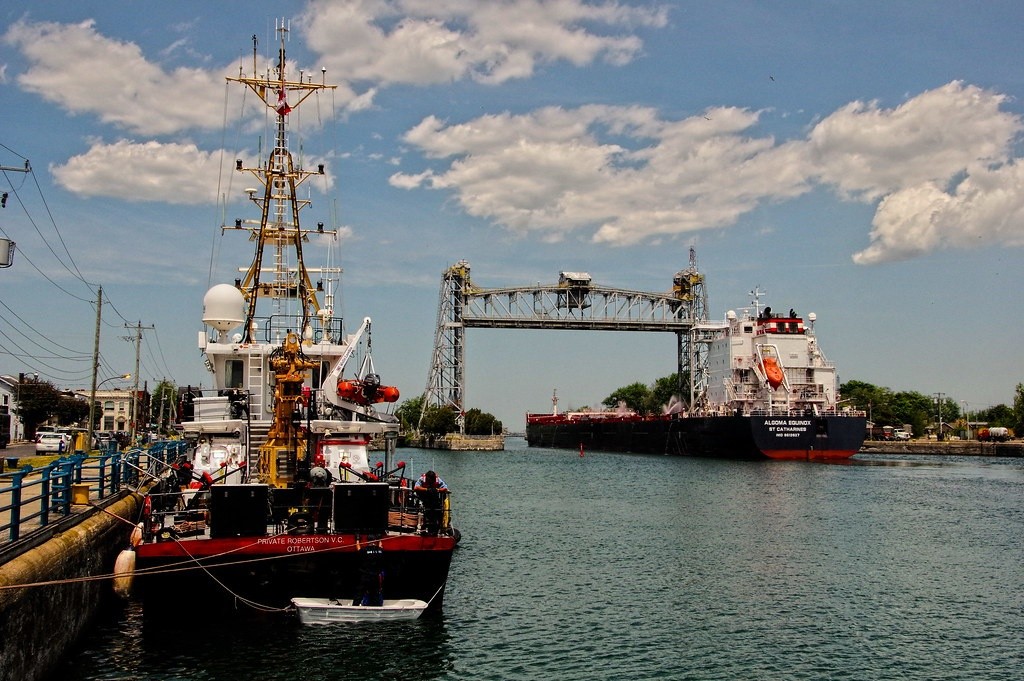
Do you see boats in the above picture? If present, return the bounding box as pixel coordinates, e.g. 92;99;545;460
291;595;427;627
129;15;460;628
525;284;870;460
758;356;784;384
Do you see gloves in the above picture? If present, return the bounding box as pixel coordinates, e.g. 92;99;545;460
426;487;439;494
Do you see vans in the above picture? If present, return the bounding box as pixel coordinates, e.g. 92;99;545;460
35;426;58;442
57;429;100;449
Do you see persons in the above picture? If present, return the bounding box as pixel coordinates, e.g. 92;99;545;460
414;471;448;535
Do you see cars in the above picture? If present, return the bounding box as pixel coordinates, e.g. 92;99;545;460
97;432;113;443
35;433;66;455
148;431;158;440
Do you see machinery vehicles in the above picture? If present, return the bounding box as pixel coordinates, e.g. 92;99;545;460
976;427;1008;441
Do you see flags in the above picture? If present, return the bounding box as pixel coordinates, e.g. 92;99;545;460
277;86;291;116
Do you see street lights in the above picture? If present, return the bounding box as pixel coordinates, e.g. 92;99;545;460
88;374;131;451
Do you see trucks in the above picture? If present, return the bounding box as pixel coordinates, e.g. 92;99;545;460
884;430;910;441
979;428;1016;440
